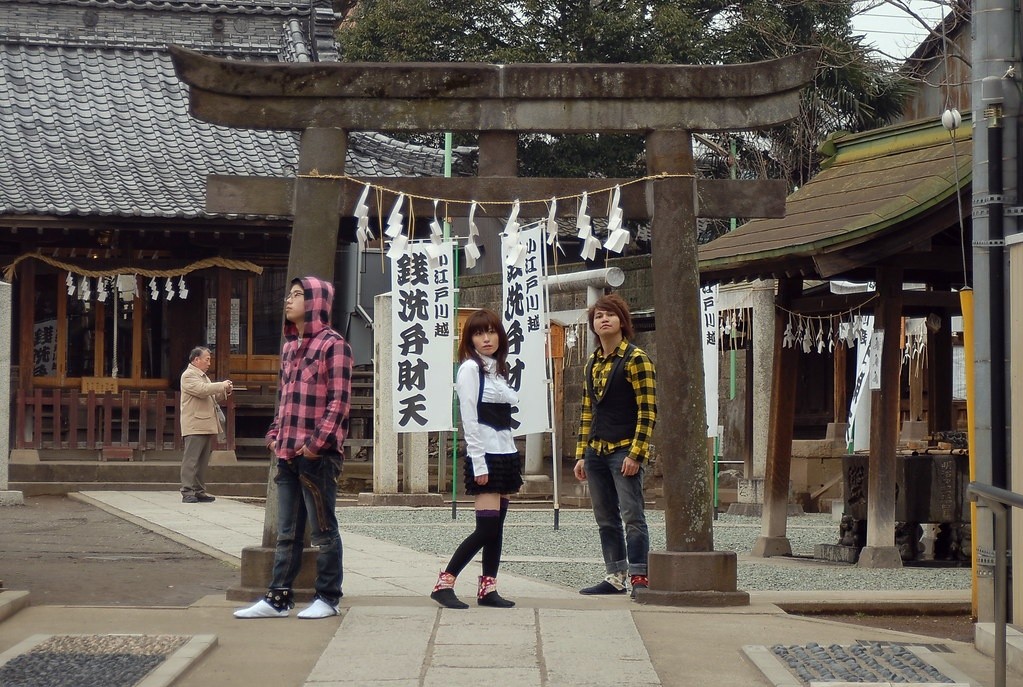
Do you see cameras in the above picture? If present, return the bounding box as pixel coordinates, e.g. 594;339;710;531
229;384;233;390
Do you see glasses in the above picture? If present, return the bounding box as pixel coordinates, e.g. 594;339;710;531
283;292;305;301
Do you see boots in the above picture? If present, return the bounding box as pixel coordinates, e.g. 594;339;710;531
477;576;515;607
431;569;469;609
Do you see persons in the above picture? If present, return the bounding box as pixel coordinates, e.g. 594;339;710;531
573;295;657;597
234;278;353;618
180;347;233;503
430;309;523;608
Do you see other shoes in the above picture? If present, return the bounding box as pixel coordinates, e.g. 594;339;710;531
630;576;648;597
233;599;290;618
297;599;341;618
579;574;627;595
183;495;197;503
195;495;215;502
